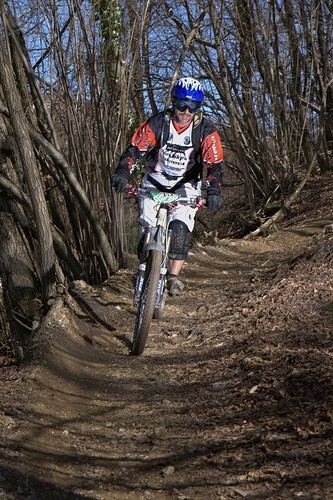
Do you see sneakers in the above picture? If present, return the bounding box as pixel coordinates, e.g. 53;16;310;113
168;276;184;295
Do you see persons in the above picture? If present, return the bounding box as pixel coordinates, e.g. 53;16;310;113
113;76;225;297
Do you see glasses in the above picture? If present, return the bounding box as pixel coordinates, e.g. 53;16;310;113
173;99;200;113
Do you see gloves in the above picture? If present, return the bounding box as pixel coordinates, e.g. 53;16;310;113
112;175;128;193
207;187;223;212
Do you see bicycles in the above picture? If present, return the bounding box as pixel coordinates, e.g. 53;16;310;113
110;182;222;356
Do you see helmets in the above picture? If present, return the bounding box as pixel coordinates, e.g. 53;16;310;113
171;77;204;102
